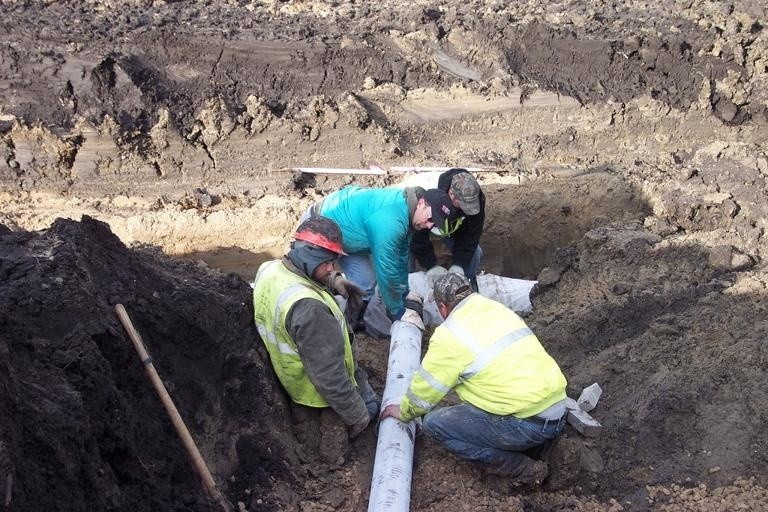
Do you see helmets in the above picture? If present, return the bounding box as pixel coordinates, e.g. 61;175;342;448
295;216;349;257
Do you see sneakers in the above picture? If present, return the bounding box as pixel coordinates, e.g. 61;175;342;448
510;459;548;488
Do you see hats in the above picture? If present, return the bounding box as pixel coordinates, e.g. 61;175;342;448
428;273;471;303
424;172;480;227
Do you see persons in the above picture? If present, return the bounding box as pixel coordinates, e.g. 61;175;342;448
398;166;486;295
300;185;452;334
380;272;569;493
252;215;382;460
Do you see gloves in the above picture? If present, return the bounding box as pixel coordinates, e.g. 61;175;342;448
402;289;424;316
334;276;368;306
350;411;370;439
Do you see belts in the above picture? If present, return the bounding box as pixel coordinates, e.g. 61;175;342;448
523;416;564;424
